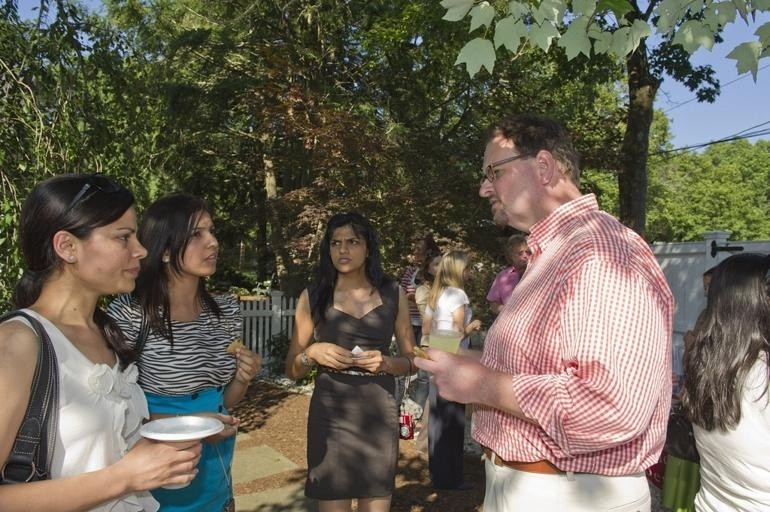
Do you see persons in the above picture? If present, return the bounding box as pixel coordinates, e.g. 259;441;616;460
102;193;262;511
410;249;444;426
485;232;533;314
422;248;483;491
671;263;717;403
0;173;203;510
412;115;679;512
283;212;420;511
677;252;770;511
399;232;441;403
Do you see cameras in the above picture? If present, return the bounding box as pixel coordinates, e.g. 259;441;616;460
399;414;416;440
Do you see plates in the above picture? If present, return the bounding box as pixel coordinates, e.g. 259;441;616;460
137;415;226;443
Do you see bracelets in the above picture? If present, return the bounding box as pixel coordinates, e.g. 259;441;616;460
176;412;180;417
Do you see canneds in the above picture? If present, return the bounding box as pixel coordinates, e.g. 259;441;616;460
398;414;413;439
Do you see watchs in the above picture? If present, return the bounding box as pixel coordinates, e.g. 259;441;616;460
301;352;312;368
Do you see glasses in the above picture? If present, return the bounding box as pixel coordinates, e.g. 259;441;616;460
64;172;123;215
483;152;533;183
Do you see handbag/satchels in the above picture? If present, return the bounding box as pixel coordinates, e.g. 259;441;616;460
661;402;701;462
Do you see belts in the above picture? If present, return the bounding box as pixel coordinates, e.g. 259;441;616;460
481;443;565;475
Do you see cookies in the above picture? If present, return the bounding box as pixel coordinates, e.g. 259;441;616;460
225;338;246;356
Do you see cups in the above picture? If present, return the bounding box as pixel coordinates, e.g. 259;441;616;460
428;328;464;355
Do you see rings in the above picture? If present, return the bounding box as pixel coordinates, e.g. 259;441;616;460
428;374;435;381
